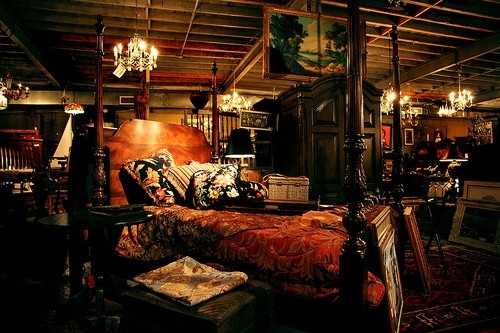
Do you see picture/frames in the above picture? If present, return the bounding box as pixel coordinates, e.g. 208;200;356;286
382;122;414;151
447;181;500;254
261;4;368;82
238;108;279;133
370;204;431;333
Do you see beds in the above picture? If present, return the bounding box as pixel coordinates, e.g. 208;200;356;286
95;118;398;333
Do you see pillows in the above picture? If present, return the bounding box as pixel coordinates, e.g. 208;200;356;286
162;160;233;209
191;165;242;208
121;149;176;208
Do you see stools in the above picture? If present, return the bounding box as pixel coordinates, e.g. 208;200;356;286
121;267;274;333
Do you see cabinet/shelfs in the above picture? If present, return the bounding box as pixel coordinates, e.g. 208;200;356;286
277;72;381;207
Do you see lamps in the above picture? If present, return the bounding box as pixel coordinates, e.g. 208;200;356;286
223;129;256;172
379;34;408;115
217;69;251;114
0;36;30;112
111;0;160;79
437;63;474;119
64;89;85;117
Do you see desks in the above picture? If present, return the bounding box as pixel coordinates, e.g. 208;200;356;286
33;205;155;333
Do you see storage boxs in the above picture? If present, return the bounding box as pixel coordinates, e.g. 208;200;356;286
264;175;311;201
